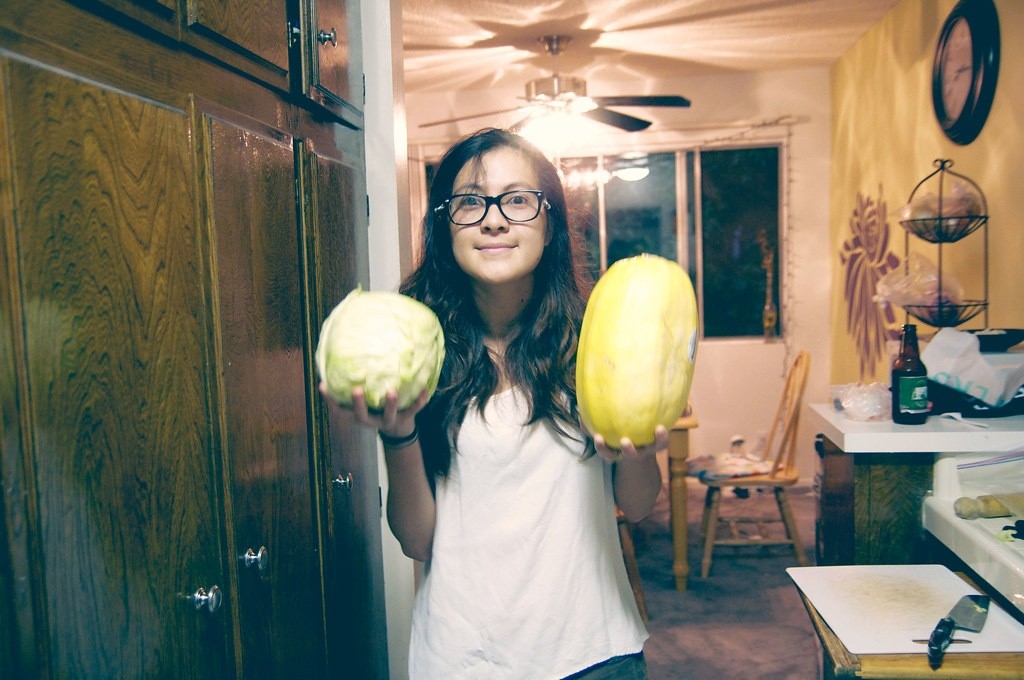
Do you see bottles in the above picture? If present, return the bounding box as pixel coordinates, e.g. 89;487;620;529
891;325;928;425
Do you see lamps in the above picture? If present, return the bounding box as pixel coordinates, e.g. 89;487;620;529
523;36;588;128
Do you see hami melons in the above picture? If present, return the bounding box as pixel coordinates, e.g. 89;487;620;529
575;253;697;448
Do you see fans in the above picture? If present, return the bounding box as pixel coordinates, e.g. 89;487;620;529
420;35;691;133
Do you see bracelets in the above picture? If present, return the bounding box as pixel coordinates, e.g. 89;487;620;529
378;428;418;451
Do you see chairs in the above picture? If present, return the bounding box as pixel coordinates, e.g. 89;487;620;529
697;351;812;579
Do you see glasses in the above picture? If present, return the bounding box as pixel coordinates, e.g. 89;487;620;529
433;190;550;225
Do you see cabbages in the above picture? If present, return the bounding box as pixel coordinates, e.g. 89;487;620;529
316;290;445;414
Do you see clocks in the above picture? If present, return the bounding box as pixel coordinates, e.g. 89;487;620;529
931;0;1002;145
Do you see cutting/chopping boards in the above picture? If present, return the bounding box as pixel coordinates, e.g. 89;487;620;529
786;564;1024;655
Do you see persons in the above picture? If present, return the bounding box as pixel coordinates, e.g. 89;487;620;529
319;127;670;680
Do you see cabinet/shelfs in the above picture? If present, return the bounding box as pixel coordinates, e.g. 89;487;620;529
900;158;991;333
0;2;394;680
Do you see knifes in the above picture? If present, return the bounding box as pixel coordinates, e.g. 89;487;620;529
929;595;991;671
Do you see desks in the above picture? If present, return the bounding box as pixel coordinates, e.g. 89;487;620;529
792;560;1024;680
662;415;700;592
